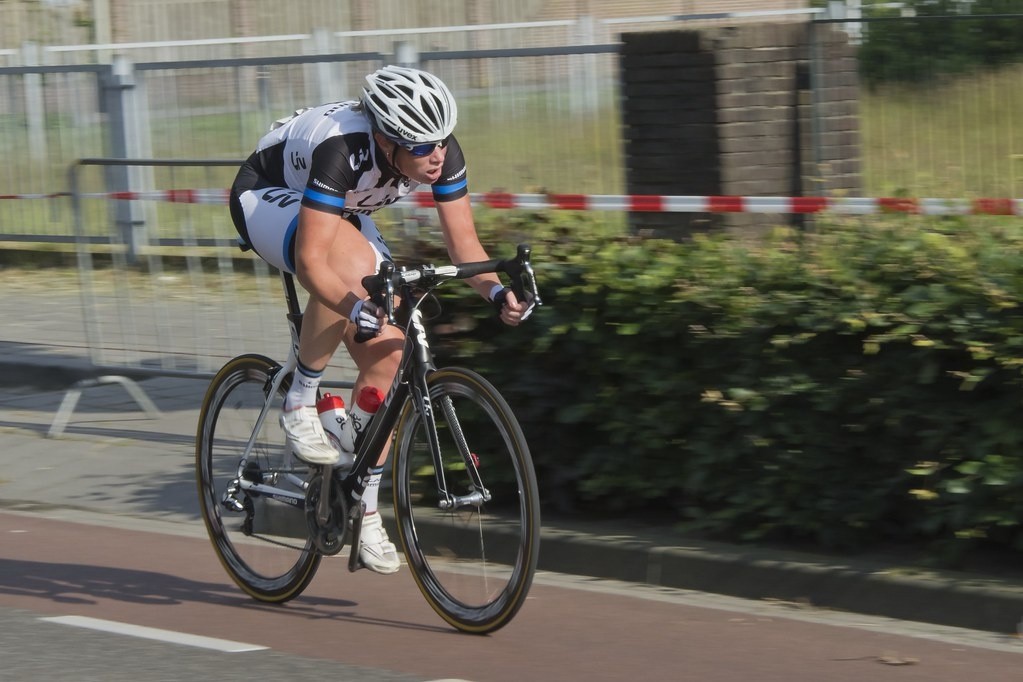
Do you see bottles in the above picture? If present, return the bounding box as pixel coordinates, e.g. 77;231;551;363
317;392;355;480
348;386;385;434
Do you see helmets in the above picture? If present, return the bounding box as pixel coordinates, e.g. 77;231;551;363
363;64;458;146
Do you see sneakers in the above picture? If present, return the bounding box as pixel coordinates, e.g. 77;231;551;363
277;396;340;466
349;510;401;575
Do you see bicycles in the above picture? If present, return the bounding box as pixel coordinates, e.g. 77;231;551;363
192;238;543;635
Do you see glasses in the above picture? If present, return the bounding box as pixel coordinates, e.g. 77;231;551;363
410;133;453;157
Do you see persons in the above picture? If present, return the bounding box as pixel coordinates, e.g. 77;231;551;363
229;64;535;574
